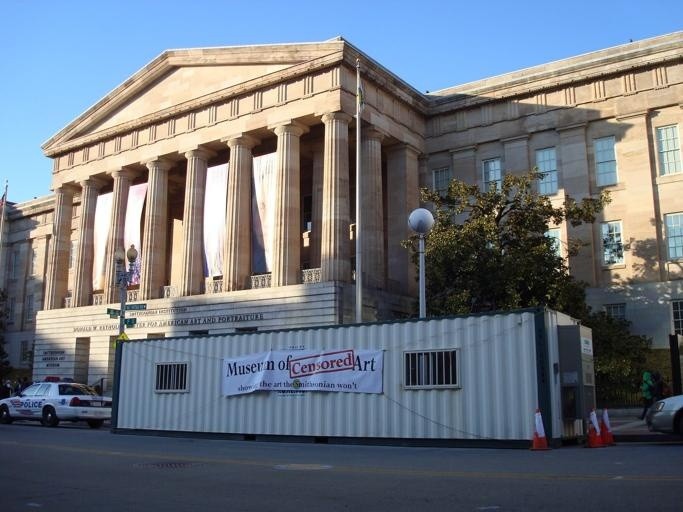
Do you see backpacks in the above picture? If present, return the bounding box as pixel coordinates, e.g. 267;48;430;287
660;382;673;396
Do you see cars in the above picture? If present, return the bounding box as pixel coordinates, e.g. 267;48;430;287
0;376;113;429
645;394;682;436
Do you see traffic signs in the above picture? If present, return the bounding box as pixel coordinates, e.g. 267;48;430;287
123;318;136;325
125;303;146;310
107;308;120;316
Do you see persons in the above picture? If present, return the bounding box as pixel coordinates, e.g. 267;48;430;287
0;375;27;398
651;371;664;403
635;367;657;420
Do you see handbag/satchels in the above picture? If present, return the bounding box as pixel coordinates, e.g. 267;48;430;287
649;384;656;395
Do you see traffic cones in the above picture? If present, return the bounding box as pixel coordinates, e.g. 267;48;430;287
600;404;616;446
583;405;607;448
529;408;551;451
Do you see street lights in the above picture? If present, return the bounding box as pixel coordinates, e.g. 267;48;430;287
113;244;139;338
407;207;434;387
